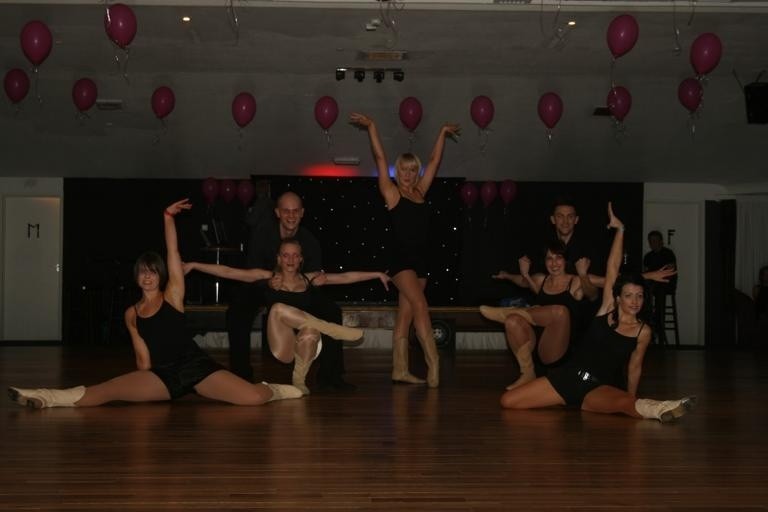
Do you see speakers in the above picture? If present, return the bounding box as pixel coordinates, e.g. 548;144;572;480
743;81;768;124
408;317;462;353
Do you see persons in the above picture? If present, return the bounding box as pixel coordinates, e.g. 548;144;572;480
477;194;698;426
8;199;303;410
751;264;768;354
349;110;464;388
183;192;396;396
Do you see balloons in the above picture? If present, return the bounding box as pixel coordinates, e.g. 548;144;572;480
400;97;423;129
538;92;563;128
471;95;494;129
459;177;517;222
232;92;256;127
106;4;137;46
608;14;639;57
21;21;52;65
152;86;175;118
679;78;703;111
608;87;632;121
200;176;254;213
4;69;29;102
315;96;338;128
690;32;720;75
73;78;97;110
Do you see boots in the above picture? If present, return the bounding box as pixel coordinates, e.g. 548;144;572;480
635;395;697;422
261;305;537;402
8;385;86;411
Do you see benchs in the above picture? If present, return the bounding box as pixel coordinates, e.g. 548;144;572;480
183;303;508;352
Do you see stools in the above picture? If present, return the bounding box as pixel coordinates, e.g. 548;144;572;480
652;285;681;350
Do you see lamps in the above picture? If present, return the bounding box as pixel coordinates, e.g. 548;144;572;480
334;66;405;84
